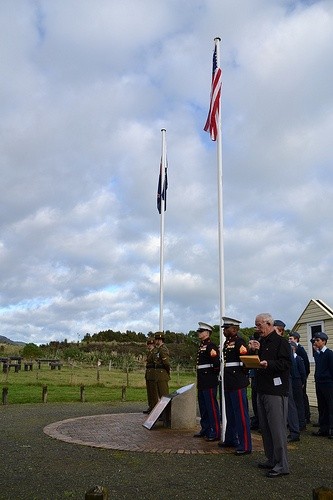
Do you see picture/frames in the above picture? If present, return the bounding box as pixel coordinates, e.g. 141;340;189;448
141;395;174;431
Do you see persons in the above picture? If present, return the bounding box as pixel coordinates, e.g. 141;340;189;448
248;312;291;476
249;337;257;419
152;331;170;421
273;320;300;442
142;341;158;415
288;331;311;423
196;321;220;441
310;332;333;439
289;341;306;430
218;316;253;456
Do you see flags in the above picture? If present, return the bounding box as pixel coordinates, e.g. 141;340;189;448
202;45;222;141
156;158;167;214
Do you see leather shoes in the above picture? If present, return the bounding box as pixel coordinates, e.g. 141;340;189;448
266;468;290;478
208;435;216;441
258;462;275;468
194;431;208;437
235;449;251;455
218;440;237;447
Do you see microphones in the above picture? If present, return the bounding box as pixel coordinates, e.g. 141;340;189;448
254;332;260;355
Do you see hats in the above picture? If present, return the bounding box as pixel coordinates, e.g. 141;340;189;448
155;332;166;339
221;316;242;328
146;338;156;344
198;321;215;331
289;332;300;338
273;320;286;327
313;332;328;340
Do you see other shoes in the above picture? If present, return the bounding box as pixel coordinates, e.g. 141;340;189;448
142;407;153;413
284;418;333;442
248;414;261;432
